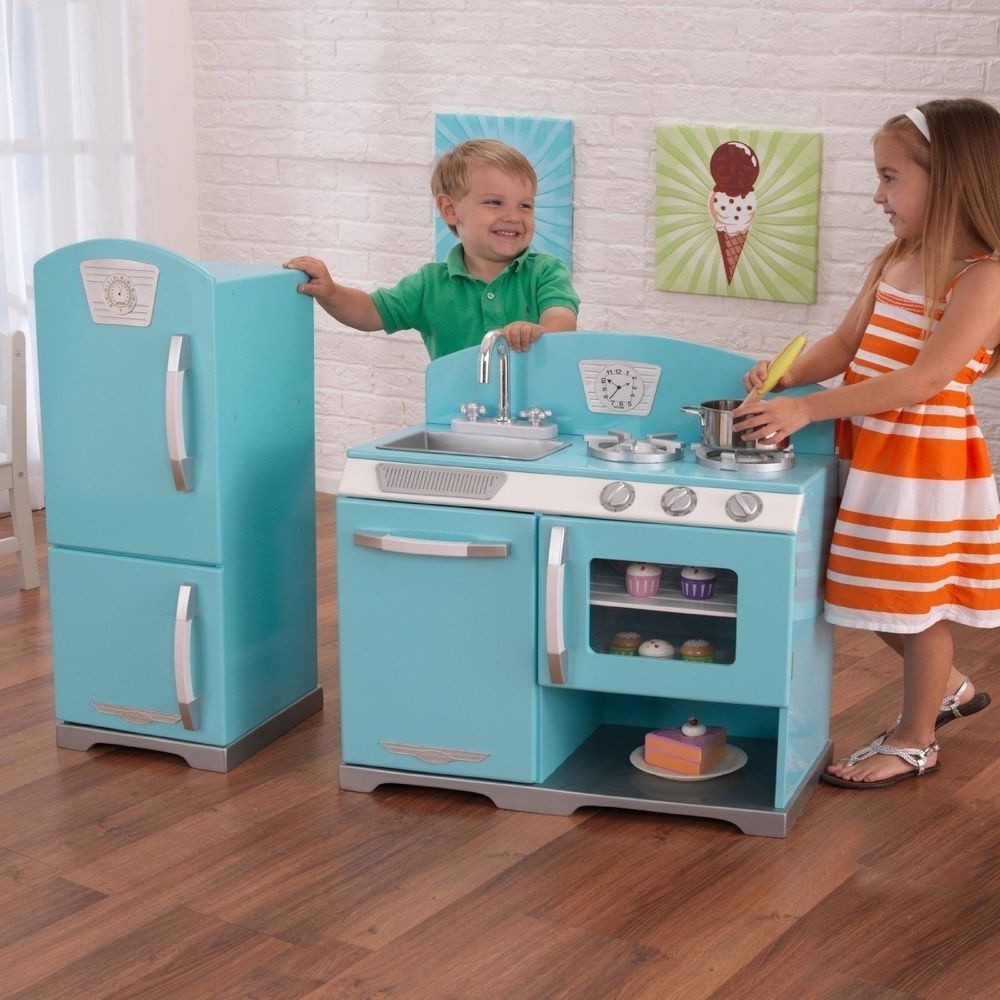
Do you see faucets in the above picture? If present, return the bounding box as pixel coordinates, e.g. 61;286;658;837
477;330;513;422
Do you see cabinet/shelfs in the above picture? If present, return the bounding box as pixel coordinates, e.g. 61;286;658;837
337;494;604;783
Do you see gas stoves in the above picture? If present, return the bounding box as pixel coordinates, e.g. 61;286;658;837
584;429;796;473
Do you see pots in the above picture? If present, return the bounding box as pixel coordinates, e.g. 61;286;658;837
678;399;789;453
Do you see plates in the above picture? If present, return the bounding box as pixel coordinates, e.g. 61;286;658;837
630;744;748;783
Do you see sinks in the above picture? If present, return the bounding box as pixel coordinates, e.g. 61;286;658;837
377;429;573;461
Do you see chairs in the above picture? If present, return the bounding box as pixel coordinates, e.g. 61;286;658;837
0;332;41;591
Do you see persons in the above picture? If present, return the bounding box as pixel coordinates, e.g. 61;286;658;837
284;138;585;362
722;96;1000;794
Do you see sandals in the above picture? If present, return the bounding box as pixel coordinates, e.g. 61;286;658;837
821;734;942;788
878;676;991;739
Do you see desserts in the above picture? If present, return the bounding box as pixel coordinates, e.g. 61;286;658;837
681;566;715;599
624;564;660;597
644;716;726;775
607;632;715;663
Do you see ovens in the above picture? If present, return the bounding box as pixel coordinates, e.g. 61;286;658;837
538;513;792;708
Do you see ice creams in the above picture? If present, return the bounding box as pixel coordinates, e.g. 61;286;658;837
709;141;761;283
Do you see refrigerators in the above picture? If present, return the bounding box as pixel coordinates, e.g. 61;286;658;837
33;238;324;773
